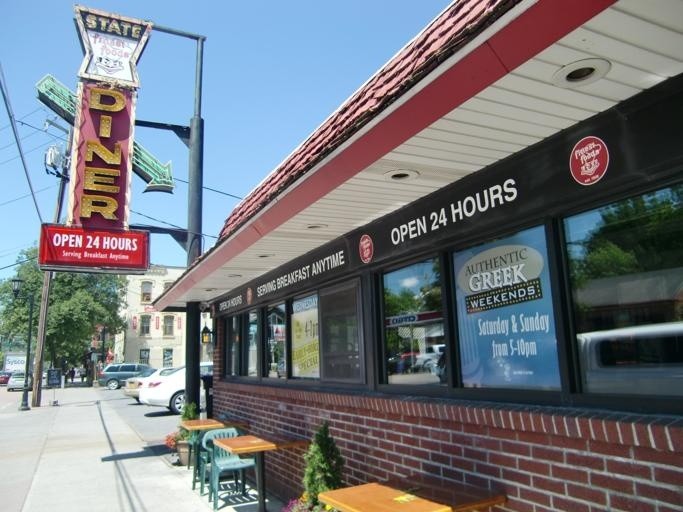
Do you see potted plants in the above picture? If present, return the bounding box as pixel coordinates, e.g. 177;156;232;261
167;402;197;465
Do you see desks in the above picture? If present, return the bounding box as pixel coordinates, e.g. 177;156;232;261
212;434;307;510
180;417;249;490
317;474;506;510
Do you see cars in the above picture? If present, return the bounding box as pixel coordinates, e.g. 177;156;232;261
96;360;214;415
0;370;48;391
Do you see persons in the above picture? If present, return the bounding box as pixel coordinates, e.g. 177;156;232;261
69;367;74;384
79;367;86;383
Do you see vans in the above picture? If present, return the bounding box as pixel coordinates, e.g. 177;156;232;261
575;321;683;404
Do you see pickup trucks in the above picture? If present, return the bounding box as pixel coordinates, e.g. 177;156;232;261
413;344;446;372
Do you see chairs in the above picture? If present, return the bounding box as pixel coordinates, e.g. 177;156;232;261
201;428;260;511
192;426;244;495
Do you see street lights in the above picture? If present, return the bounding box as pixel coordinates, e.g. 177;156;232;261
10;271;33;410
